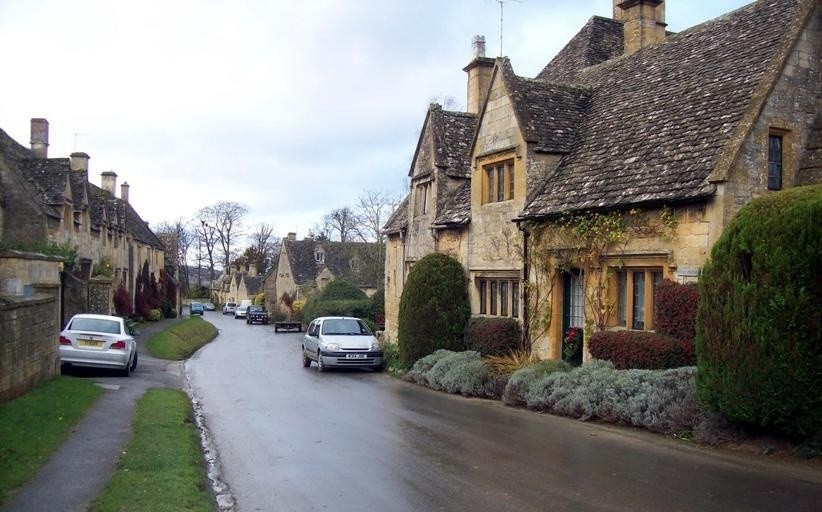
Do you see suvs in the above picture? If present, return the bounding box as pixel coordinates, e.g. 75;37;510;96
189;302;204;315
246;305;268;325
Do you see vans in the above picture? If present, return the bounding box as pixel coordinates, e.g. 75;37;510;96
223;302;237;315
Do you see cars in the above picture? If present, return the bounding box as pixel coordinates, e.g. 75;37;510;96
301;316;384;372
204;302;216;311
56;313;138;376
235;307;246;319
